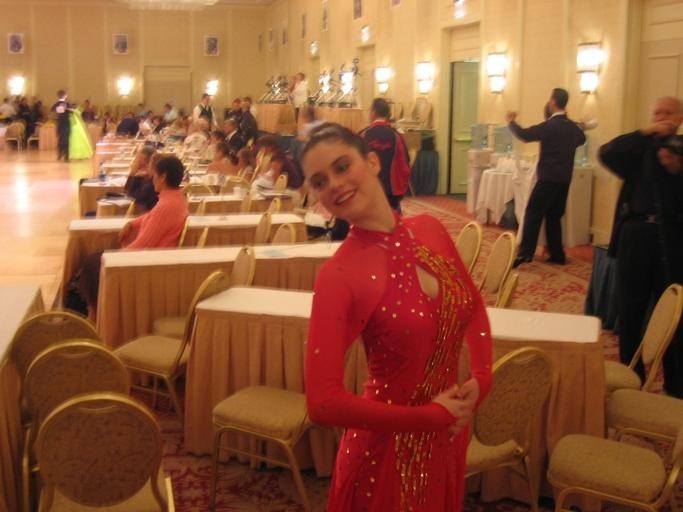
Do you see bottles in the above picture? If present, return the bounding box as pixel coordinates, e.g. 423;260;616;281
124;133;271;201
98;163;107;186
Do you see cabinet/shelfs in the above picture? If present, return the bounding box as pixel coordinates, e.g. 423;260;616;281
516;165;590;249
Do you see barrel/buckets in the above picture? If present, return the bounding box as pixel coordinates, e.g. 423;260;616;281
469;123;488;151
573;137;589;166
493;127;512;153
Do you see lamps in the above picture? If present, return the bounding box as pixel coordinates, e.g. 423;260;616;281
373;40;609;99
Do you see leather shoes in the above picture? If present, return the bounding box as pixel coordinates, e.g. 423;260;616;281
545;255;566;266
510;256;533;269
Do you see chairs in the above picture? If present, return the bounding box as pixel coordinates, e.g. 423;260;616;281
12;311;98;378
80;128;303;216
153;244;256;410
607;386;682;460
547;427;682;511
210;346;343;511
21;338;132;511
253;214;270;244
177;219;190;248
111;270;228;425
196;226;209;249
462;346;553;512
455;221;482;274
0;119;102;151
478;231;518;310
497;274;518;310
270;224;296;243
37;390;175;511
605;283;681;389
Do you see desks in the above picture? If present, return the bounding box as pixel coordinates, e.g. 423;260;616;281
53;213;307;308
97;242;343;347
0;285;46;512
185;286;604;510
476;168;526;227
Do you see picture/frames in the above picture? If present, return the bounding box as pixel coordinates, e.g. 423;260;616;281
202;33;220;57
254;0;403;55
6;30;26;55
111;32;130;55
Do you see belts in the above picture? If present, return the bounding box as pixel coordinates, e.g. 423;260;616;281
625;210;664;227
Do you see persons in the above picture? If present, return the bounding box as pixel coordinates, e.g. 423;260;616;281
509;103;598;256
299;121;495;510
122;148;160;210
287;73;309;122
67;153;188;325
356;98;410;217
505;88;586;269
597;96;681;401
